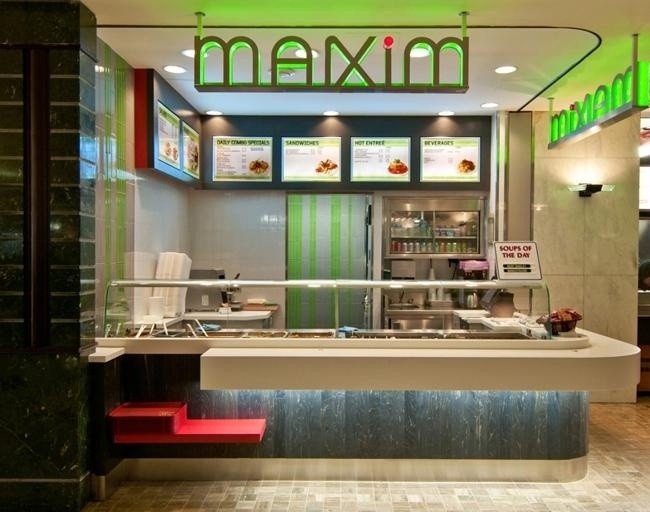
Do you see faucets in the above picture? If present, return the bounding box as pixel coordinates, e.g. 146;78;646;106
399;290;404;302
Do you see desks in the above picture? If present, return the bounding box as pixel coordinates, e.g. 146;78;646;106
135;303;279;331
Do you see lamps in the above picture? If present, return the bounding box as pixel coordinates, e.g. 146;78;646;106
568;182;615;196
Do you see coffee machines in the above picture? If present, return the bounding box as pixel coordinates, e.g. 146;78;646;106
457;261;489;309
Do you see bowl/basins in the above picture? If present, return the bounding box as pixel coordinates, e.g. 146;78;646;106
537;319;578;336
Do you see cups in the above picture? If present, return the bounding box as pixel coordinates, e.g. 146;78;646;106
428;268;452;300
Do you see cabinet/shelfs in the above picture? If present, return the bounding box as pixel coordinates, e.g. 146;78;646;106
381;195;486;260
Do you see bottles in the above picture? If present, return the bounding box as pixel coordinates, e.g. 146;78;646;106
435;221;477;236
390;218;432;236
390;241;477;253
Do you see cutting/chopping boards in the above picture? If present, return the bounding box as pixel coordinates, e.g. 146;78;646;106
241;304;280;311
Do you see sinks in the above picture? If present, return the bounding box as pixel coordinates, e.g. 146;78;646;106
390;303;419;311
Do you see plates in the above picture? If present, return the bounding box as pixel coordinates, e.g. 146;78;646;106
142;297;163;322
249;160;478;176
